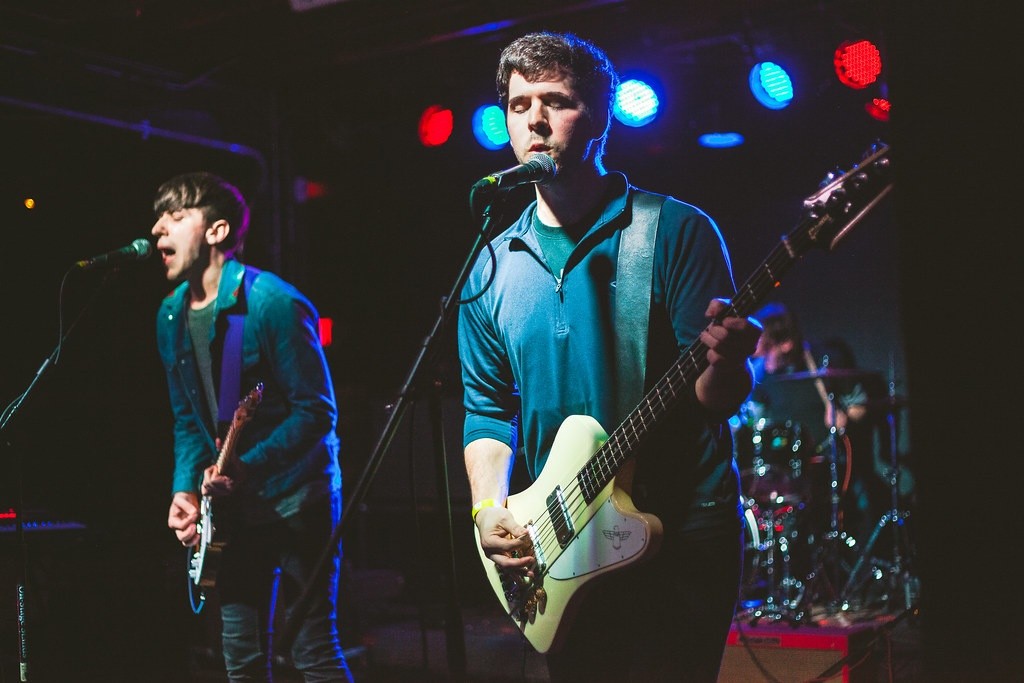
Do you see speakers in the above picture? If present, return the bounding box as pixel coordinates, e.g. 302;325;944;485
717;622;883;683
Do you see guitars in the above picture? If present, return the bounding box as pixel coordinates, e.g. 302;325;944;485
473;136;897;656
186;380;265;615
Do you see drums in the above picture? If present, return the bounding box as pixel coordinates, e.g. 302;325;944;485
736;414;815;492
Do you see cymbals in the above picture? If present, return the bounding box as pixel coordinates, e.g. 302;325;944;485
777;366;873;382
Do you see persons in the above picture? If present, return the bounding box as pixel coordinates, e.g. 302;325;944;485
456;31;766;683
728;313;870;610
151;171;354;683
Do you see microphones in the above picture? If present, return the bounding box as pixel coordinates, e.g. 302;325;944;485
75;238;152;270
472;154;557;192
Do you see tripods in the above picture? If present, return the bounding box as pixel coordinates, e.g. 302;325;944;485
733;357;916;629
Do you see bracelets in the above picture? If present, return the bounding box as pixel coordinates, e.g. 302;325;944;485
472;499;506;522
